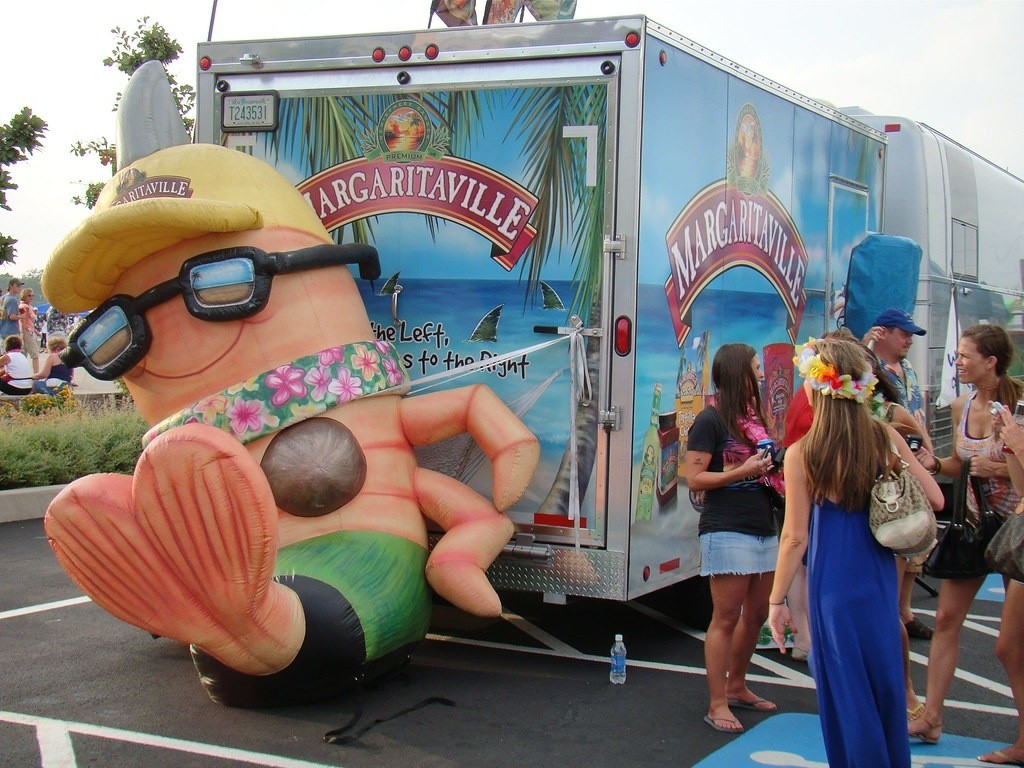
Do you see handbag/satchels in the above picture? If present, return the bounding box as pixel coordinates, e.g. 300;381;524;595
772;492;786;542
869;443;936;557
924;461;1004;580
984;498;1024;581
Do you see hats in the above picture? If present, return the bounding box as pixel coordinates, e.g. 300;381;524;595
875;308;927;336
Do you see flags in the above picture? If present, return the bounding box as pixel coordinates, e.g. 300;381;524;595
432;0;577;27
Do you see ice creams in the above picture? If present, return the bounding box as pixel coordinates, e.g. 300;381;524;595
33;307;38;315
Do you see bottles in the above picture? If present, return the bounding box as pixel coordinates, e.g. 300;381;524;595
1002;399;1024;455
609;634;628;684
633;383;679;523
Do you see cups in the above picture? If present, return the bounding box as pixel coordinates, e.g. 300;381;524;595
762;343;796;451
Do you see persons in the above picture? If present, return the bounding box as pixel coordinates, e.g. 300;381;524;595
0;278;25;356
33;307;40;338
977;401;1024;765
864;347;931;721
32;337;74;394
772;337;945;768
778;328;858;661
40;322;47;347
18;287;39;374
686;344;779;732
45;305;69;340
908;325;1024;744
861;307;934;640
73;313;82;326
0;335;33;395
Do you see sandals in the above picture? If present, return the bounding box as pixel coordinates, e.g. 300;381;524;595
908;718;943;744
979;749;1024;766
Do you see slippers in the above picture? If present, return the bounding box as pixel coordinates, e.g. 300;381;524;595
727;695;777;711
704;715;744;732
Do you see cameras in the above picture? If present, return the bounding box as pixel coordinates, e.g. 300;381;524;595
990;401;1004;416
904;434;924;452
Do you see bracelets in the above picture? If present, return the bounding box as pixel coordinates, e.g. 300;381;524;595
928;456;941;475
770;602;784;605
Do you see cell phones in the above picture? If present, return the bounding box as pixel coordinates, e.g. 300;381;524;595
760;445;771;460
1001;400;1024;455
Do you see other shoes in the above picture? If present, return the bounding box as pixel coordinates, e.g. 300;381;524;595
906;702;925;721
906;618;934;639
792;646;809;661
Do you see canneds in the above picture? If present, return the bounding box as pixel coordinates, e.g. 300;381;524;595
756;438;775;462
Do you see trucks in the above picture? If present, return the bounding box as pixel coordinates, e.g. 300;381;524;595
196;14;888;628
